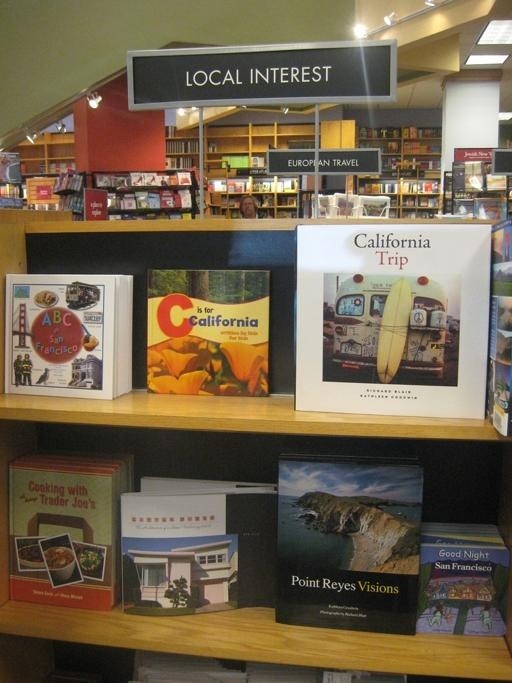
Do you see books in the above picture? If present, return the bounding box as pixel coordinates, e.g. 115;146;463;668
402;211;433;219
322;667;406;683
401;181;437;194
108;212;193;221
382;158;400;172
64;195;85;221
248;156;265;168
0;152;23;185
244;661;322;683
402;127;441;138
166;125;176;137
39;161;46;171
165;140;200;153
360;141;399;153
276;448;423;636
9;448;156;611
6;274;133;400
54;172;82;194
48;670;106;683
403;196;440;208
363;182;398;194
221;156;251;168
276;177;298;192
209;141;217;151
1;183;22;200
402;140;440;154
206;178;228;192
146;270;271;398
401;158;435;172
276;195;296;219
131;650;245;683
390;197;398;219
19;161;26;175
296;223;490;420
165;156;194;169
485;218;511;440
49;161;77;173
443;161;511;219
251;179;275;192
414;521;508;636
358;127;401;139
226;178;251;192
119;476;277;617
105;189;192;209
94;171;192;186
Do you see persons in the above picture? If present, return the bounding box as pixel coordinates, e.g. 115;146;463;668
239;194;268;220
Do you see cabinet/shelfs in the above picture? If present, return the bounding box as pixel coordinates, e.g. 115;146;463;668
97;168;200;220
9;132;75;176
26;171;87;221
443;169;512;221
0;206;512;683
164;121;355;219
357;125;441;218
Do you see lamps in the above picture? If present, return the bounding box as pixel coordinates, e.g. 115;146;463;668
87;91;103;109
385;12;400;25
